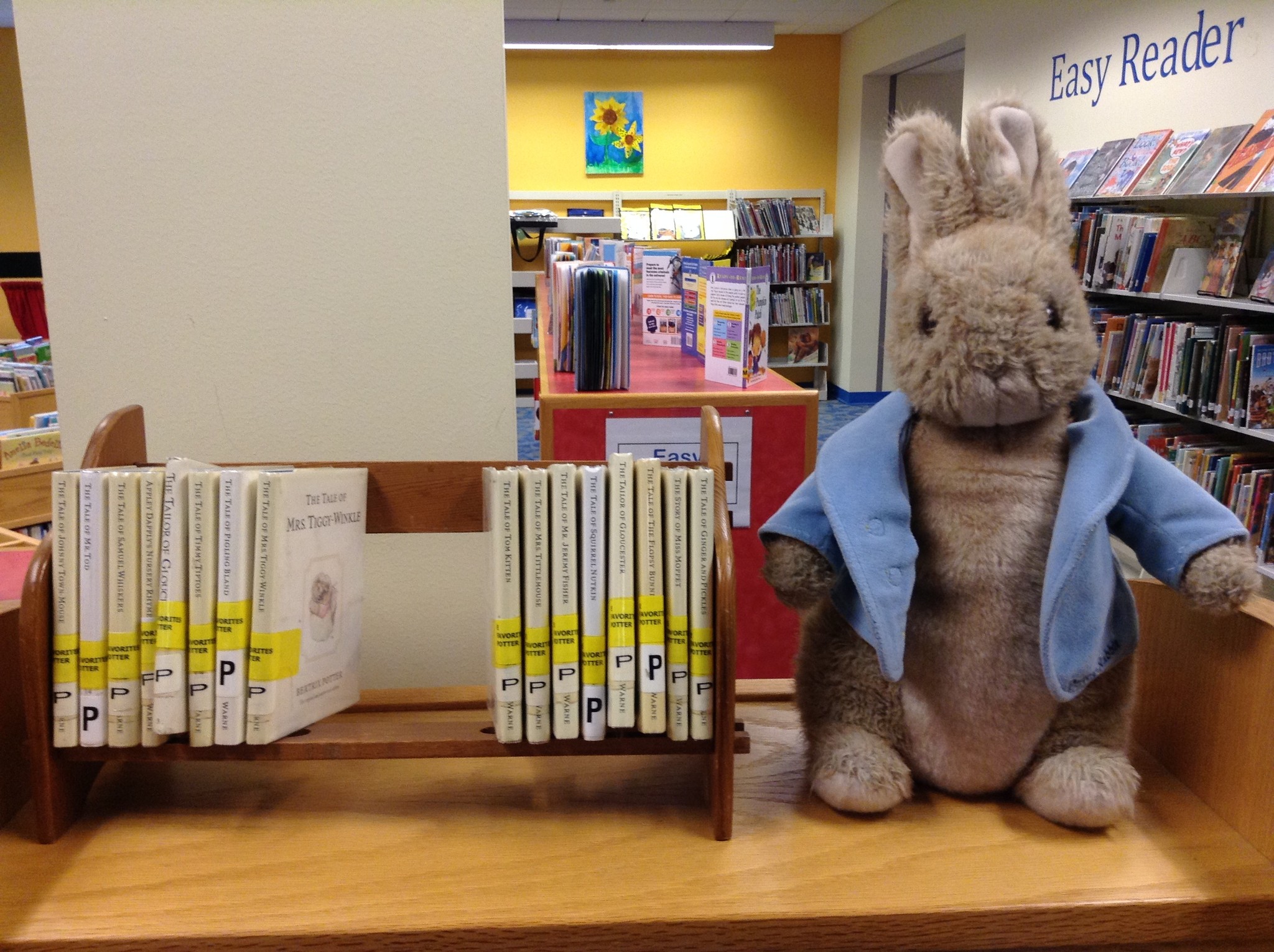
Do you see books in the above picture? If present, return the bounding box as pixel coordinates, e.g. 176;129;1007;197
0;336;54;395
52;456;368;747
620;203;736;241
544;260;769;393
1057;108;1274;564
0;411;60;438
788;327;819;363
482;453;714;743
730;197;825;325
15;524;52;540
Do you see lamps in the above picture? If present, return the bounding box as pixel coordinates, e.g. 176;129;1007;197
504;19;774;49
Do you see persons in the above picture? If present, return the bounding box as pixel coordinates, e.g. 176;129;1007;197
1263;386;1272;394
1257;266;1274;297
794;333;818;363
1198;242;1220;289
1267;394;1272;405
1214;243;1234;295
1262;377;1272;390
1250;385;1265;407
1170;131;1245;194
1206;242;1227;292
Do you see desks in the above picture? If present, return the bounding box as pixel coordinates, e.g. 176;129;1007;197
0;384;1274;952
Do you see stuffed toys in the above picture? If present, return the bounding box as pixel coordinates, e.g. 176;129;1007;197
757;85;1260;830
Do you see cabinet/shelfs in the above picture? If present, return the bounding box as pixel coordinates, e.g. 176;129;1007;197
1071;192;1274;599
536;272;820;696
507;190;618;411
617;190;833;401
